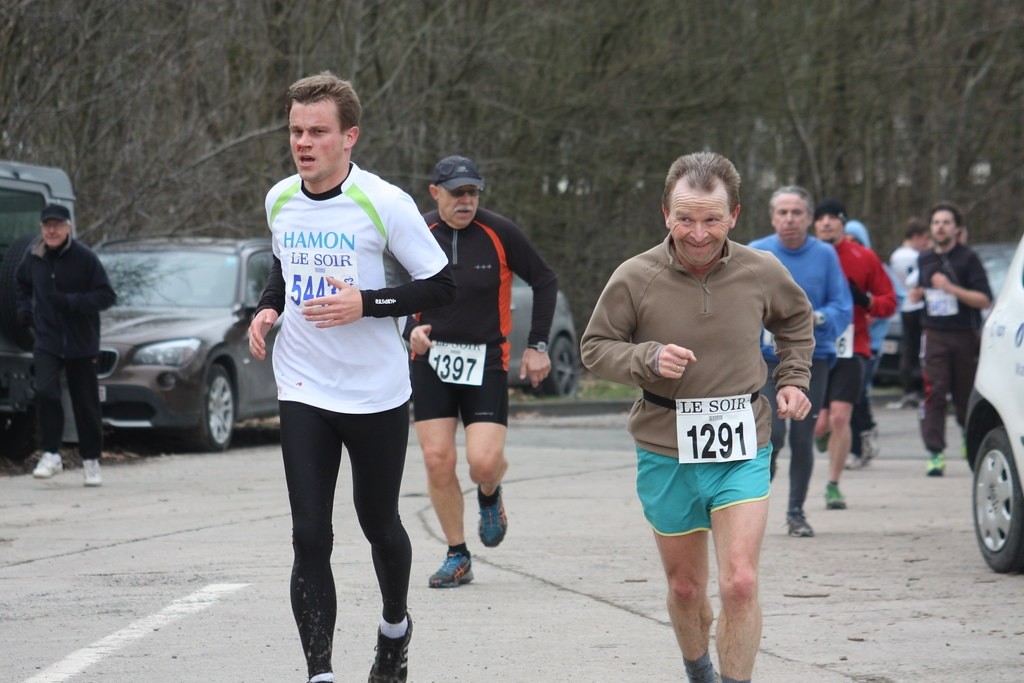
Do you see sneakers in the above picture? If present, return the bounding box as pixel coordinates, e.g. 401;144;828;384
787;510;814;537
428;550;473;588
33;451;61;478
826;485;846;509
81;458;102;487
477;483;507;547
814;435;827;453
367;613;413;683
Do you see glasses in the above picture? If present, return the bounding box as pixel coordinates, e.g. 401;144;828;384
439;184;481;197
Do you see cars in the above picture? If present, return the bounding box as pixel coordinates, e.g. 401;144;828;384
869;238;1018;393
960;226;1024;576
83;233;282;454
508;271;581;401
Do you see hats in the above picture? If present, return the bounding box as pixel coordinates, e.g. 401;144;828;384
41;204;69;221
815;201;848;228
433;155;485;191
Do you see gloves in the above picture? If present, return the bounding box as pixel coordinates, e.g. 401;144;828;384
18;310;34;329
49;293;68;311
847;277;869;308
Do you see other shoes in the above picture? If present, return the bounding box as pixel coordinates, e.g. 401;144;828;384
846;429;879;469
926;453;945;477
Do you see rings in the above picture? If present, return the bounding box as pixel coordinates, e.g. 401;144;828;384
798;410;802;414
677;366;679;370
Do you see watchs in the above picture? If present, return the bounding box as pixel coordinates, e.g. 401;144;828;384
528;341;548;352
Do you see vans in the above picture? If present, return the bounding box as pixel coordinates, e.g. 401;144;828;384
0;159;81;467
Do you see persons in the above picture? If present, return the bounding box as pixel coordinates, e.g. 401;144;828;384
14;206;117;486
383;156;558;589
910;200;993;476
743;185;853;538
580;151;816;683
813;194;897;469
846;218;904;432
248;70;459;683
890;218;969;407
813;323;860;508
